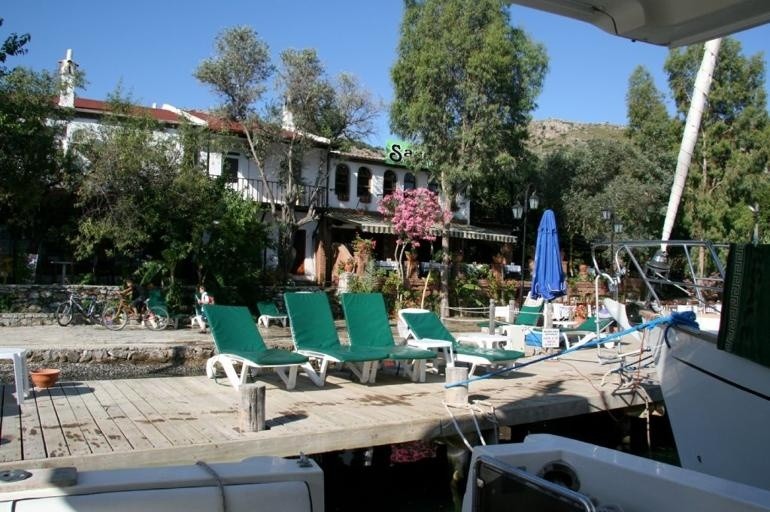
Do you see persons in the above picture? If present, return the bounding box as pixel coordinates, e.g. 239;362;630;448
196;285;211;335
117;278;149;324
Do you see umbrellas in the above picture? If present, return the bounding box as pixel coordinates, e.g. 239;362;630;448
529;208;569;329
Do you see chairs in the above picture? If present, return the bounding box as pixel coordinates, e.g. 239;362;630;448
476;294;545;328
201;291;525;391
533;316;614;349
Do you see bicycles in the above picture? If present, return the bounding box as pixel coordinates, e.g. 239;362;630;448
57;290;169;331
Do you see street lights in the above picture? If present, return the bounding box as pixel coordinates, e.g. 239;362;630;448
601;198;624;277
512;183;540;311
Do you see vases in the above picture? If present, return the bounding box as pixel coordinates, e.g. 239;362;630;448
29;368;60;388
